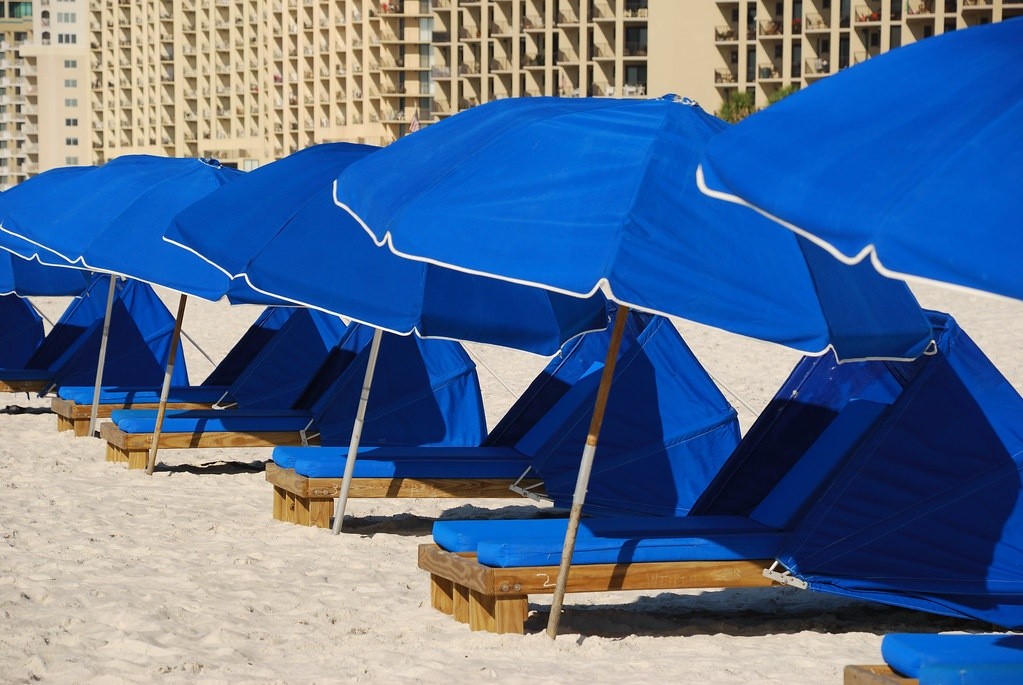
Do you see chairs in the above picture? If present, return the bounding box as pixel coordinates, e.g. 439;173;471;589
0;274;1023;685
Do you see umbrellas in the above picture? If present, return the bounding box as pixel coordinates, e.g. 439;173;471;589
164;142;610;534
0;166;118;439
333;94;937;638
0;158;305;473
695;15;1023;304
